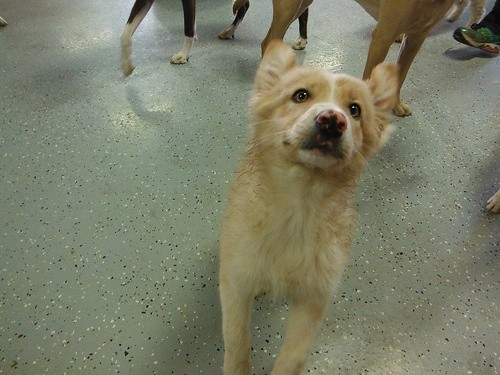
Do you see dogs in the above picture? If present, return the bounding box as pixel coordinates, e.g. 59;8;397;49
260;1;454;117
395;0;487;51
218;36;402;374
118;0;311;77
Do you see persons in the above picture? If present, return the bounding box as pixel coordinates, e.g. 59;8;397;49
453;0;500;54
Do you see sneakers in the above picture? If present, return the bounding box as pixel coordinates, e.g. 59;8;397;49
454;22;500;54
